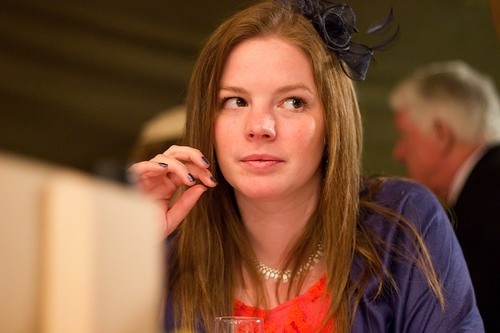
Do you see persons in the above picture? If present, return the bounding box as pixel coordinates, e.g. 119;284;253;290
389;59;500;333
122;0;484;333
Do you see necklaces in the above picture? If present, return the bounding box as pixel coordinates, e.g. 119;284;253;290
251;239;326;283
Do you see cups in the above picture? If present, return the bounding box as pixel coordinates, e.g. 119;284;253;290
213;316;264;333
